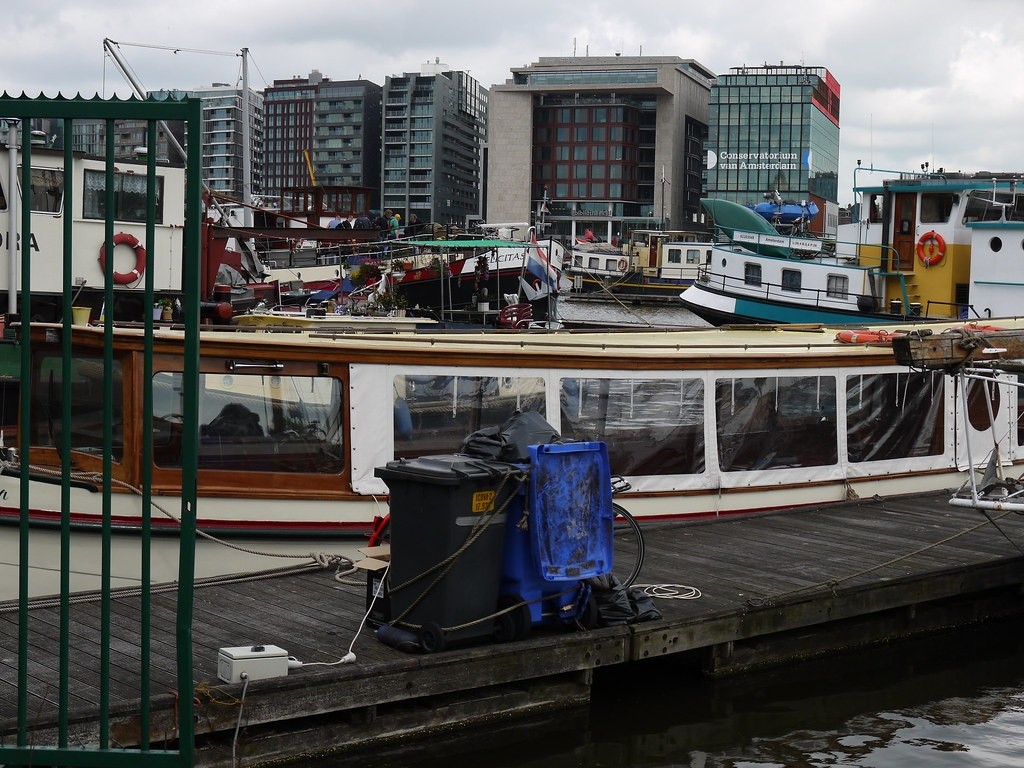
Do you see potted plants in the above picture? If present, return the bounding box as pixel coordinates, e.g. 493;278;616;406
401;258;415;269
396;295;408;317
391;258;406;282
478;295;491;311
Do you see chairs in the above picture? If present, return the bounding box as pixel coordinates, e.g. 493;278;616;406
495;303;534;330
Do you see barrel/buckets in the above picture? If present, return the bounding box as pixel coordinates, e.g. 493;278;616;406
891;301;901;314
910;303;921;316
72;307;92;326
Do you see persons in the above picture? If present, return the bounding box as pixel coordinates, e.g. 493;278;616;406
373;209;401;260
353;211;372;229
335;215;353;229
611;236;617;247
327;215;341;229
404;214;424;236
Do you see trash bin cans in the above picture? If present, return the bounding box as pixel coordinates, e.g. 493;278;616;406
373;454;529;654
498;442;616;642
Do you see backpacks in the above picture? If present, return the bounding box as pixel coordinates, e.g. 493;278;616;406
372;216;384;230
386;218;395;230
335;221;348;229
353;217;366;229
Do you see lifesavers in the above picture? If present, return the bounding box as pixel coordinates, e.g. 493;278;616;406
949;324;1006;332
617;259;628;271
917;230;946;266
836;330;907;344
100;233;146;285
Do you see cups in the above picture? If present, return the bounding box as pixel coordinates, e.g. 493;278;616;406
299;289;303;294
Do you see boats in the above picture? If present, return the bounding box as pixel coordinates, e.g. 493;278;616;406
615;242;738;299
212;237;565;316
0;314;1024;539
569;234;641;279
682;162;1024;328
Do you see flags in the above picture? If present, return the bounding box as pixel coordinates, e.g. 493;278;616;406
526;232;556;283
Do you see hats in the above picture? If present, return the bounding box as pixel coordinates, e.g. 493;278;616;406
395;214;402;218
